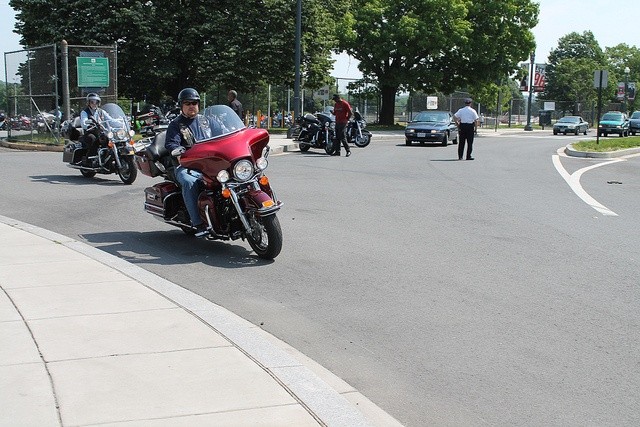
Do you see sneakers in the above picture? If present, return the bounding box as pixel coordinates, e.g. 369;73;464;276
346;152;351;156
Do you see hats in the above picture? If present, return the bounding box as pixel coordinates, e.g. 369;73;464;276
332;95;339;100
464;98;472;103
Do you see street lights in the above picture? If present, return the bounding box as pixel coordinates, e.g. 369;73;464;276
625;67;631;110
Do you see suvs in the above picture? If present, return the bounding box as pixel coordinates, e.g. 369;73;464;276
629;111;640;135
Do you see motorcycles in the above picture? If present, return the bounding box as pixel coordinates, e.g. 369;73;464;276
346;112;372;147
59;103;137;183
122;105;284;258
292;114;336;154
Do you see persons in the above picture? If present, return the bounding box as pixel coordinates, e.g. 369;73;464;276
330;94;353;157
165;87;224;240
453;98;479;160
480;112;485;128
227;90;243;121
80;92;112;168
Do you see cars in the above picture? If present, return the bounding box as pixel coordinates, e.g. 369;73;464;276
405;111;457;145
599;111;628;137
553;116;588;135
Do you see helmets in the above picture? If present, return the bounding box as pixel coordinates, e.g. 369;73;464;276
87;93;101;106
178;88;200;107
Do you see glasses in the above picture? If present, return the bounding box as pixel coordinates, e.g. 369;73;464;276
181;101;199;106
